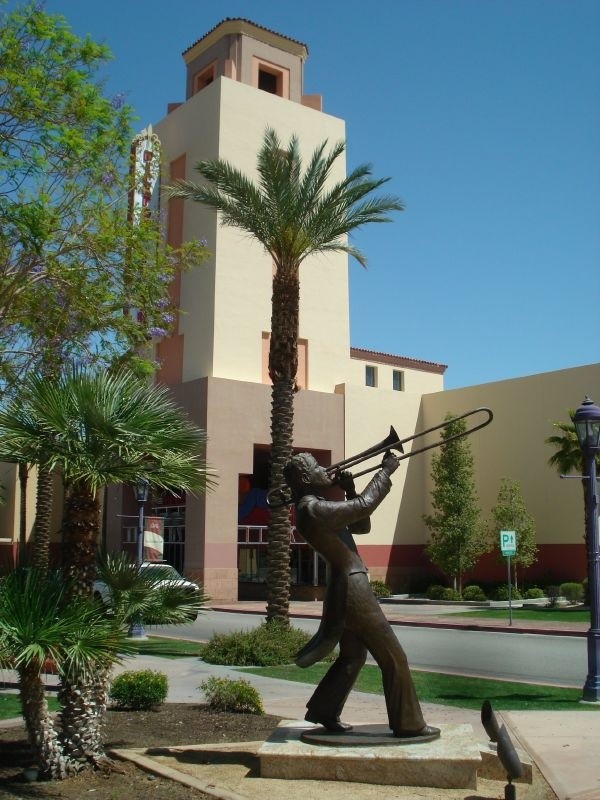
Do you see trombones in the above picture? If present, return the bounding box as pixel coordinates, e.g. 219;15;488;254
264;406;495;508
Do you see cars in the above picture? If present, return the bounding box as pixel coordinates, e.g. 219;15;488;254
92;563;202;623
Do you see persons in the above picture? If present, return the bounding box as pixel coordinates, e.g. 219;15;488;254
283;452;441;737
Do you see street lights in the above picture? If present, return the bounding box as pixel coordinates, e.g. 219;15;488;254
128;476;153;639
571;393;600;704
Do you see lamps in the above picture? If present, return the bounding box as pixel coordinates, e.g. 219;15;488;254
481;700;501;748
496;723;522;800
